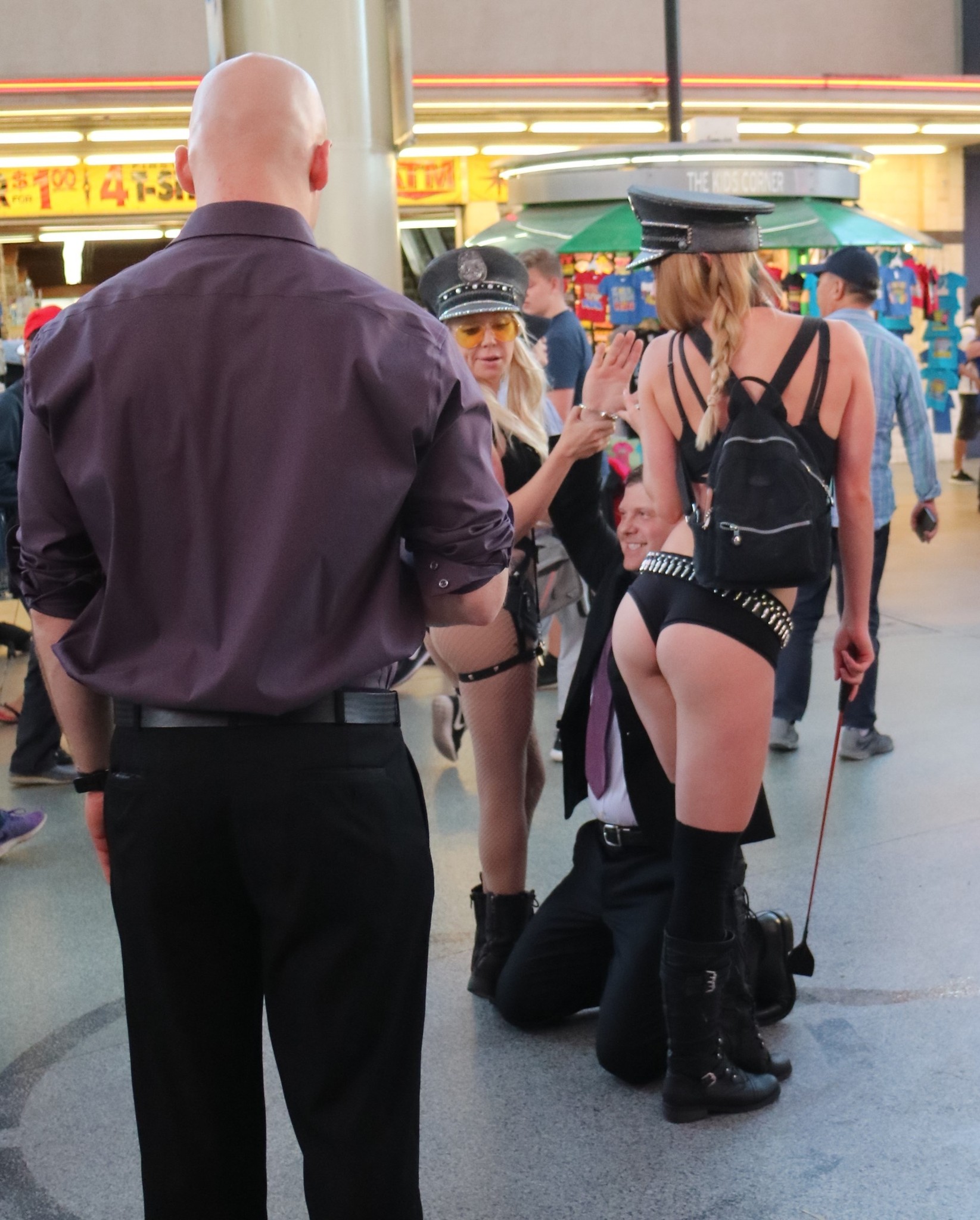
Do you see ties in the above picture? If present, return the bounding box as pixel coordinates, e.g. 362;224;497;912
585;630;612;800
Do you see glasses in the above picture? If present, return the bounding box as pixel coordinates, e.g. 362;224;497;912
449;319;518;348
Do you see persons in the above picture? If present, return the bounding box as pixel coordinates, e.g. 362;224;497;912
766;247;942;764
2;304;76;860
497;327;799;1032
515;247;644;690
21;51;513;1220
418;245;615;1000
950;297;980;481
593;189;877;1128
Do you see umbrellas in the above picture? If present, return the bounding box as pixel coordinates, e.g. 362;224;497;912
555;194;946;249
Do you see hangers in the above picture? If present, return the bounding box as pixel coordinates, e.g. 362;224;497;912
938;280;950;296
888;246;903;270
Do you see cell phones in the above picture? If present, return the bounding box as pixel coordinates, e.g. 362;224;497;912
915;507;936;542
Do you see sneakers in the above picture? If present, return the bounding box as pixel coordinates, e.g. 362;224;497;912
0;807;47;858
432;696;465;762
768;718;800;750
839;727;894;760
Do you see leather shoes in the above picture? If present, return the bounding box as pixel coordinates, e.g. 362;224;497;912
753;910;796;1025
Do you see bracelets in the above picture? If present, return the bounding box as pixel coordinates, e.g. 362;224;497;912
75;767;111;794
577;402;618;423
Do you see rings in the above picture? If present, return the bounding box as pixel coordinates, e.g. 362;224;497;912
634;404;640;410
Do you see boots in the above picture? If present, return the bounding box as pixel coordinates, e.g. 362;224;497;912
717;884;793;1082
467;879;540;999
660;925;779;1122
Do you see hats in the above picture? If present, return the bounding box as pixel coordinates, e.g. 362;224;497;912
626;186;775;270
419;246;529;321
24;306;62;345
798;247;879;290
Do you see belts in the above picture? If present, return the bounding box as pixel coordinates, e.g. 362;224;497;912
594;821;639;847
113;693;397;728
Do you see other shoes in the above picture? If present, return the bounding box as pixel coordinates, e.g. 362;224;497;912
391;646;430;686
8;765;74;784
949;471;975;484
533;661;557;689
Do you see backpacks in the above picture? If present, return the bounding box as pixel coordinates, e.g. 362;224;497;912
674;319;832;590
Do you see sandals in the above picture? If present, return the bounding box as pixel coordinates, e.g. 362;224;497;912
0;702;20;724
